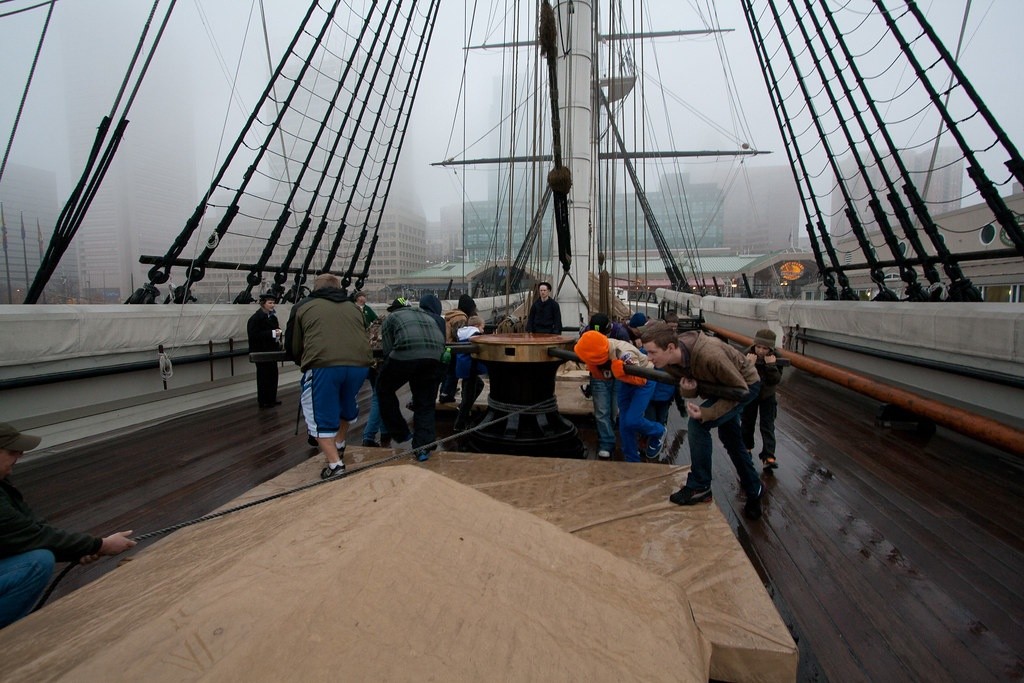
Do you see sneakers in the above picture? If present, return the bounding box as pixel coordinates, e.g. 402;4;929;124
337;444;346;459
742;484;764;521
320;464;346;478
670;485;712;505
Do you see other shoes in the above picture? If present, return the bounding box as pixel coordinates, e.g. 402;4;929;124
415;449;428;462
390;438;414;449
439;395;456;404
381;434;393;448
454;423;469;431
645;427;668;459
406;401;415;411
457;404;472;417
599;450;610;457
762;457;778;468
363;440;380;447
308;434;319;447
579;384;593;401
259;400;283;408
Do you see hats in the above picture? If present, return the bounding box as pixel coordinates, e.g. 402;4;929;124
386;297;412;313
353;292;365;299
628;312;647;328
458;294;477;313
574;330;610;366
260;294;276;301
754;329;777;356
0;421;41;451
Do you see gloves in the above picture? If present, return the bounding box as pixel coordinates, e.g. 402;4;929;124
585;363;602;378
612;359;646;385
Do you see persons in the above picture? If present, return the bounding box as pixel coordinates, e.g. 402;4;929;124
439;295;476;403
284;274;373;479
377;307;446;461
575;311;767;519
247;294;282;408
362;297;412;449
453;316;487;431
525;282;562;335
0;423;138;627
354;292;379;328
406;295;446;411
741;329;784;463
